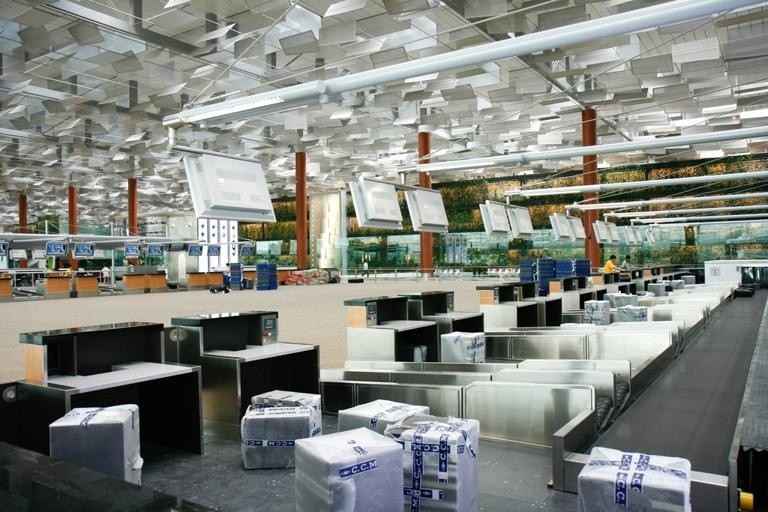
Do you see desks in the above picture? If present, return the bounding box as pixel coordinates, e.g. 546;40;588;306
620;271;650;292
648;268;668;281
342;294;441;362
475;285;539;328
502;282;562;327
549;279;592;313
654;267;673;281
17;321;205;457
575;276;607;301
396;291;484;335
634;270;657;284
587;273;628;295
170;311;321;424
606;272;636;296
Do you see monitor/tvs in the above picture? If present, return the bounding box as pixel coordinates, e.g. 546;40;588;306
348;180;404;231
549;215;571;241
633;229;642;245
479;200;510;236
0;242;8;256
45;241;68;256
207;245;221;256
506;204;533;235
147;244;162;255
404;186;449;233
124;243;142;256
75;242;94;256
623;228;635;246
569;217;586;240
182;153;277;224
606;222;620;241
646;231;655;246
591;222;611;244
188;245;203;256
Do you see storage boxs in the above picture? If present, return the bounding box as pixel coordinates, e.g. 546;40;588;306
255;263;278;291
239;405;316;469
670;280;683;289
573;259;590;276
616;305;647;322
583;300;609;325
337;399;429;436
383;412;479;512
603;292;626;308
613;295;638;308
576;447;690;512
440;332;485;363
680;276;694;284
647;283;664;297
285;268;329;285
48;404;143;488
229;262;243;291
250;390;322;438
294;427;403;512
517;259;537;282
537;258;556;295
555;259;573;280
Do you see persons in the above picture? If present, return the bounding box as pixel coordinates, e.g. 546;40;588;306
621;255;631;273
128;261;134;272
225;263;231;275
603;255;619;273
362;260;370;279
101;263;111;285
